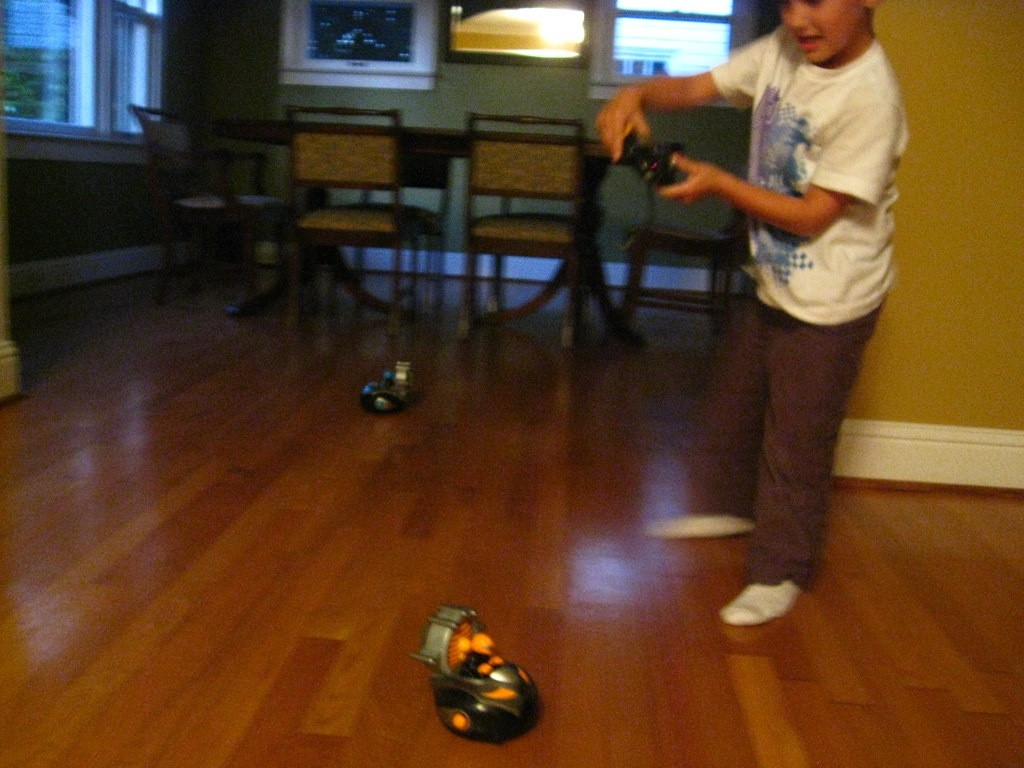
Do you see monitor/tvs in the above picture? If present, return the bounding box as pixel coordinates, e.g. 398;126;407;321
279;1;440;77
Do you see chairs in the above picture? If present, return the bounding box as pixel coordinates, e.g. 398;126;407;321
614;106;758;342
279;103;440;336
128;105;278;292
452;110;601;349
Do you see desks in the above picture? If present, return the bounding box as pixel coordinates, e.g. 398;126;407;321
211;114;628;330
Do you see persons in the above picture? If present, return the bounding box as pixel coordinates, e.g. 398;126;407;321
595;0;909;626
